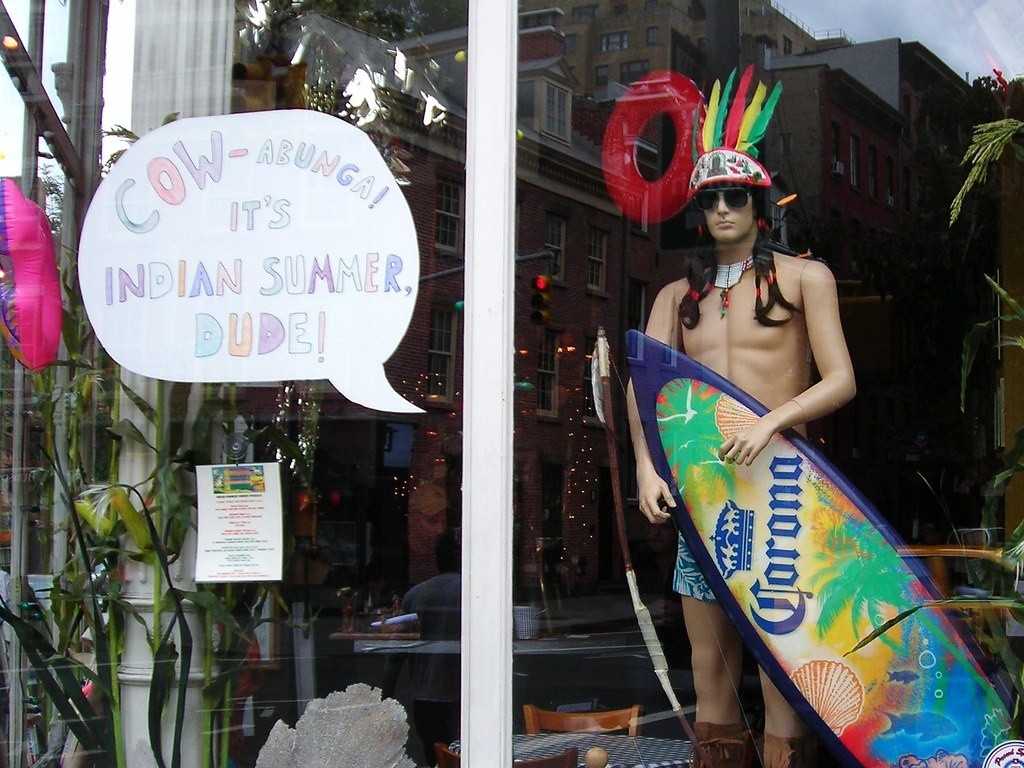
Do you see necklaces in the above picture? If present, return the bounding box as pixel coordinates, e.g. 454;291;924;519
715;255;753;317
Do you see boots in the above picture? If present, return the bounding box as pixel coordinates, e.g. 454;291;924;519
687;722;751;768
764;731;814;768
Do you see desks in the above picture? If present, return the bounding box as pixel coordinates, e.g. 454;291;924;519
449;734;693;768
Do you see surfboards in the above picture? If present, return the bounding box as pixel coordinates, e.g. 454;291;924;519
626;326;1023;768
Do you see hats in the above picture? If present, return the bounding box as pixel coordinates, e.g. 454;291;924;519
687;68;783;200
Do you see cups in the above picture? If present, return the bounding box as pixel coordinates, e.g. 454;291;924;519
513;605;542;640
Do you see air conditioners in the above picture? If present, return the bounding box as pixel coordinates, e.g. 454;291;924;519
886;195;894;207
832;161;844;174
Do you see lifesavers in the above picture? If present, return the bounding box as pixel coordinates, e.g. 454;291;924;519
601;69;709;225
0;177;64;370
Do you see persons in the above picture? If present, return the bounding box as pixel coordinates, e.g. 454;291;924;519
620;513;683;626
382;531;461;768
360;484;452;612
627;65;857;768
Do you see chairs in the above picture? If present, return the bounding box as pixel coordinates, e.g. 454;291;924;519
433;742;578;768
523;702;643;736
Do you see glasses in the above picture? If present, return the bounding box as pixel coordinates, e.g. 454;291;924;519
692;187;750;211
